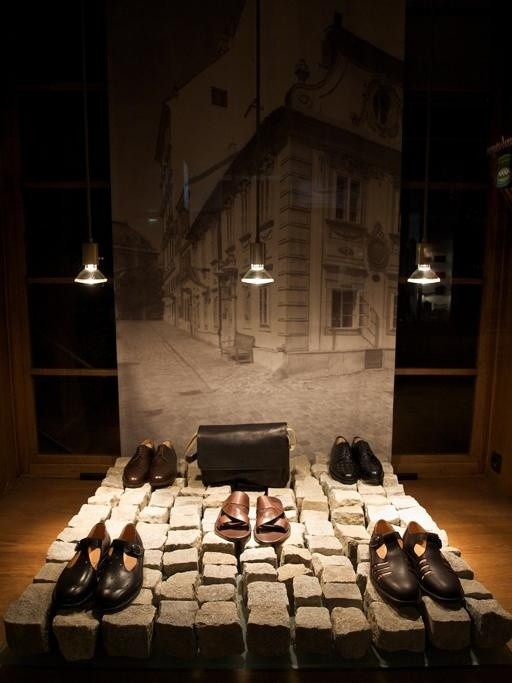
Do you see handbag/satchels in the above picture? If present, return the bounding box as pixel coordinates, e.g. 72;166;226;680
197;419;292;491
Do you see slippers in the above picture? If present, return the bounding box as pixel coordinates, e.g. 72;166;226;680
214;490;252;542
254;495;291;545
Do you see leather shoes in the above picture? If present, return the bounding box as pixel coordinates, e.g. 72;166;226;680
148;439;178;487
368;518;423;605
329;434;357;485
50;520;110;606
122;435;156;487
400;520;465;605
350;436;387;484
94;522;147;613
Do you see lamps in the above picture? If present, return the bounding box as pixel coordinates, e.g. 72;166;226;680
74;0;108;286
407;0;442;287
240;1;274;286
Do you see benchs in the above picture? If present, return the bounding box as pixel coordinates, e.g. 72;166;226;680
222;331;255;365
